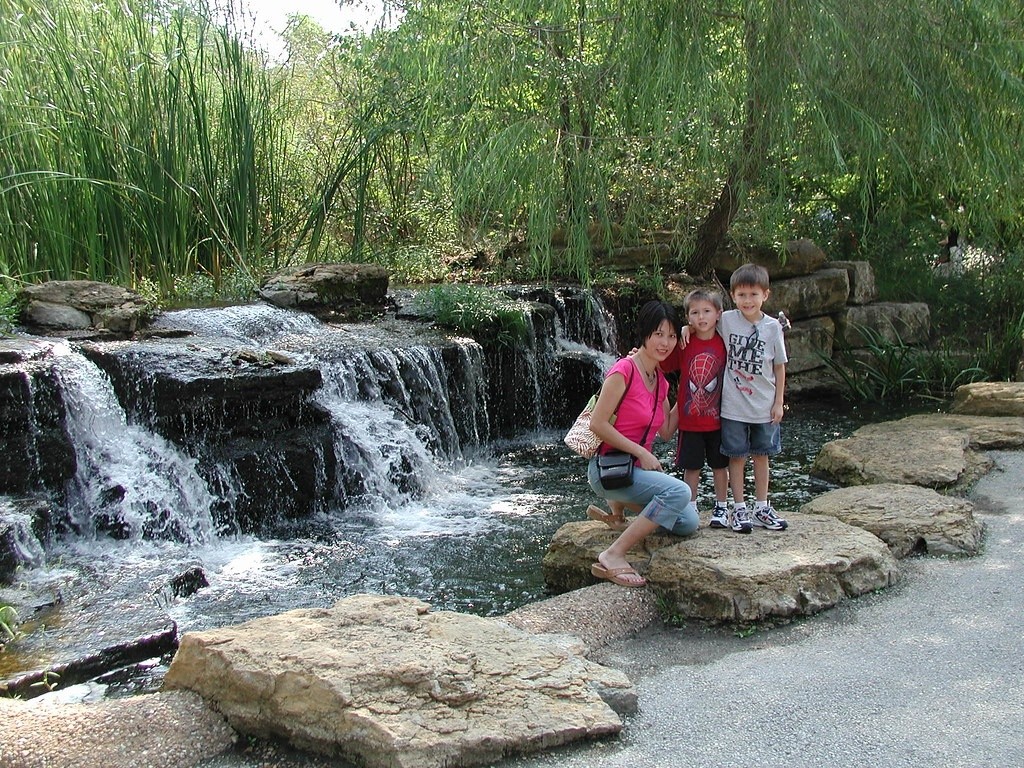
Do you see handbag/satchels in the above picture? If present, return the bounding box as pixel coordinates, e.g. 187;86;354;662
563;358;634;459
596;451;634;490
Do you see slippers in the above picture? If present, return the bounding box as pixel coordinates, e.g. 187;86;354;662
591;563;646;587
586;503;631;532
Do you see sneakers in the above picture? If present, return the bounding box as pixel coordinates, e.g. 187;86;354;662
750;500;789;530
728;506;753;533
709;501;729;528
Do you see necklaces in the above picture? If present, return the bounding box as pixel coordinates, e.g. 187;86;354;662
637;352;656;385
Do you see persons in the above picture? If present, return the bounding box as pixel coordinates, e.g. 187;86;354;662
679;264;789;534
627;289;791;529
586;301;699;587
938;220;959;276
